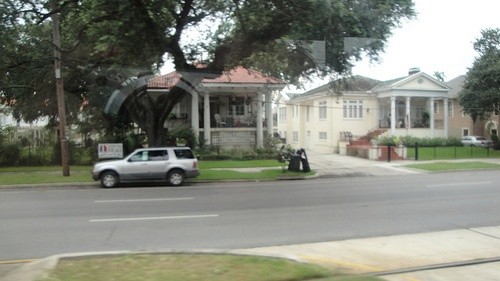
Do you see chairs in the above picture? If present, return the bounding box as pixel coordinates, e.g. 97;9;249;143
214;114;226;128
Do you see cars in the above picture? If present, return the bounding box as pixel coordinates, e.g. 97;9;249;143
461;136;494;148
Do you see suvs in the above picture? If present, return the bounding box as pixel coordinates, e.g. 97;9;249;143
91;147;201;187
